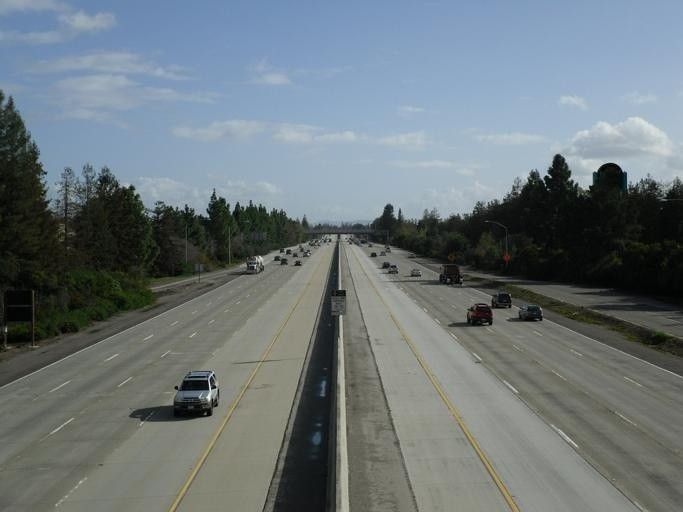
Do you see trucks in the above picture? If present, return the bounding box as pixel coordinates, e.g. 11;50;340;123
439;264;463;286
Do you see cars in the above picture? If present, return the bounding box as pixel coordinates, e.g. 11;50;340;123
380;250;386;256
271;236;332;267
388;264;399;273
370;252;376;257
406;252;416;257
384;242;390;248
383;262;390;269
385;247;391;253
411;268;421;277
516;303;545;323
344;236;372;248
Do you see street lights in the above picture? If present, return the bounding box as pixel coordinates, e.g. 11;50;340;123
483;219;509;273
227;220;250;265
185;218;212;265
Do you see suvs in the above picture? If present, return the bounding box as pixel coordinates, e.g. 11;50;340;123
466;303;493;326
490;293;513;310
172;370;220;416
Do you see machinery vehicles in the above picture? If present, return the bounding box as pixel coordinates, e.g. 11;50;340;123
245;254;264;274
